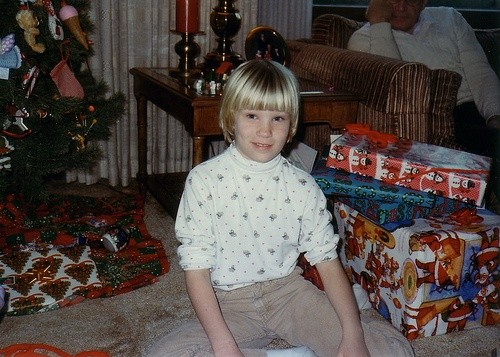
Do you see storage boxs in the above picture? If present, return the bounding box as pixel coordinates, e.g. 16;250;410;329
326;125;492;208
334;203;500;340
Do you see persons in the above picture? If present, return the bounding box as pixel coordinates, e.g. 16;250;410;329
346;0;500;215
146;57;415;357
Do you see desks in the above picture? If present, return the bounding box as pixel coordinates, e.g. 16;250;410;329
130;65;362;224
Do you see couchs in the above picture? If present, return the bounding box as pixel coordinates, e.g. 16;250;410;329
284;13;462;148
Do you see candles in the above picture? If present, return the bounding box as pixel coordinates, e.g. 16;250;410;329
176;0;200;31
